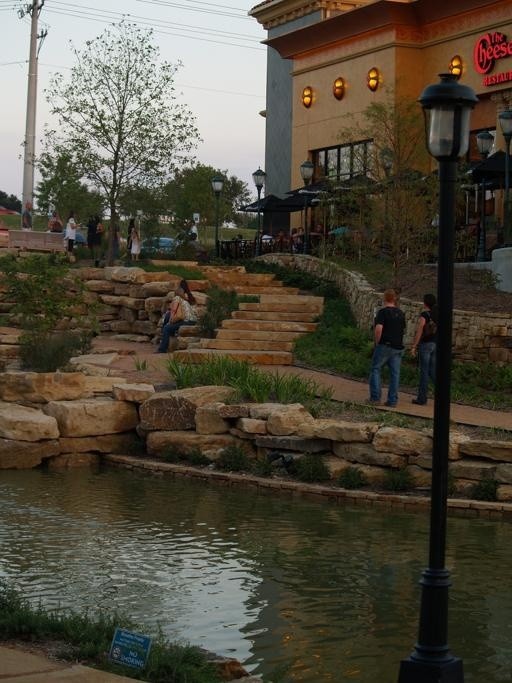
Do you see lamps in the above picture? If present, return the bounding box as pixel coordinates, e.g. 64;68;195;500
302;87;313;108
448;55;463;78
333;77;344;101
367;67;378;92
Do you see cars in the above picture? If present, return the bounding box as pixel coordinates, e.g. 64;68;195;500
73;233;89;248
141;236;208;256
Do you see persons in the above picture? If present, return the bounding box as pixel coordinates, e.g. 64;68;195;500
154;280;196;353
424;201;440;227
322;527;424;644
21;201;32;231
366;289;438;406
468;214;483;235
232;223;364;259
49;208;140;261
176;219;198;245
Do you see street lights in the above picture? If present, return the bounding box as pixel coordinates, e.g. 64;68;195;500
399;73;478;683
208;173;223;256
253;166;266;257
300;158;315;255
379;106;511;262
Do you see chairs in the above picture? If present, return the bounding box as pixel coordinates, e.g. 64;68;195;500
215;230;336;259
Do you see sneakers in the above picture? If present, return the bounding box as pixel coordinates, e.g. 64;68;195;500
365;398;396;407
411;398;426;405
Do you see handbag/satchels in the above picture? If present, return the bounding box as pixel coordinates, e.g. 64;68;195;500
424;311;437;339
170;296;186;324
